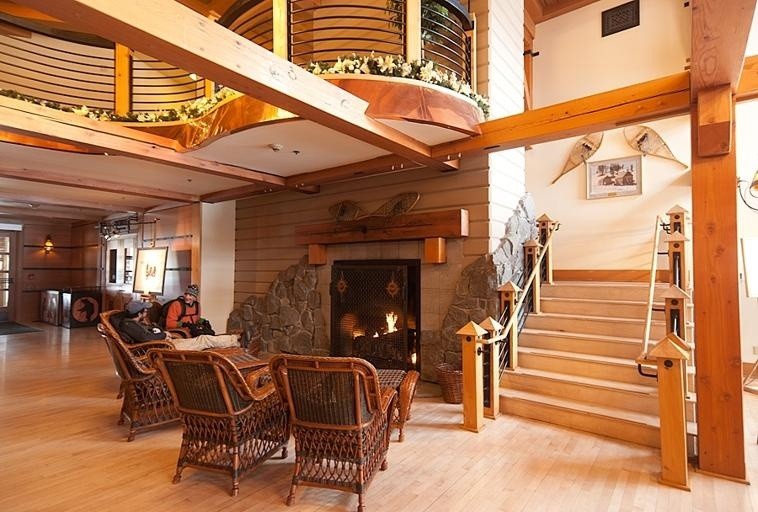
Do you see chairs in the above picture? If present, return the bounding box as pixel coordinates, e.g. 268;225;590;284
153;347;292;497
268;352;399;511
96;300;192;443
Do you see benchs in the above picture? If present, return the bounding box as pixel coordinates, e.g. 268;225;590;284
245;370;420;443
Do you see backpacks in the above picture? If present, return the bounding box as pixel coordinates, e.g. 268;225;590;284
157;298;198;328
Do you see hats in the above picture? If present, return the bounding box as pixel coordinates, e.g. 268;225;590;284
125;300;153;314
184;284;199;298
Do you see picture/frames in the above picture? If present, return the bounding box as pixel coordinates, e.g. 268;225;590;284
584;154;643;200
133;246;170;296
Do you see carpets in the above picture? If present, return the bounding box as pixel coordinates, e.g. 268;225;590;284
0;319;45;335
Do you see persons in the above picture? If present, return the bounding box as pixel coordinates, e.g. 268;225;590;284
121;299;251;352
158;284;202;338
